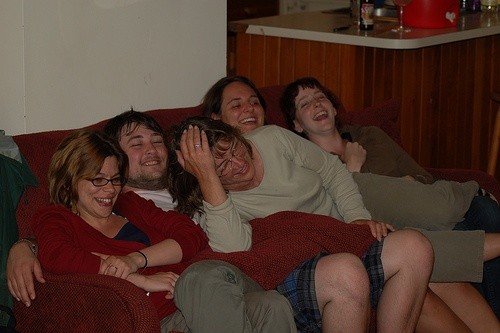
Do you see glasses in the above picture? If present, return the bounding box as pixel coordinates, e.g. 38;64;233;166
84;177;128;187
218;137;242;179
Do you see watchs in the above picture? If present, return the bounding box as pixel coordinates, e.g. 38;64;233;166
12;238;38;258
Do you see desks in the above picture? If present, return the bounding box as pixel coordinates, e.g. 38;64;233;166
230;4;500;174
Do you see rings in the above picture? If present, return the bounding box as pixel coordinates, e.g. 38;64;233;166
194;144;201;147
112;265;118;270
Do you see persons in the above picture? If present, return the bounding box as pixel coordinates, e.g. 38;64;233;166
165;115;500;283
0;75;500;333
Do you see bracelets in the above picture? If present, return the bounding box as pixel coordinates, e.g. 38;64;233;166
134;250;148;270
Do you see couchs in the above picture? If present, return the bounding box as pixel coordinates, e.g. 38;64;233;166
0;85;293;333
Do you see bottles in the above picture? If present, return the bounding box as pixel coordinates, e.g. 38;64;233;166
360;0;374;30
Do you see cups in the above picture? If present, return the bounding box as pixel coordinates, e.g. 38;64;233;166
393;0;460;27
481;0;498;15
